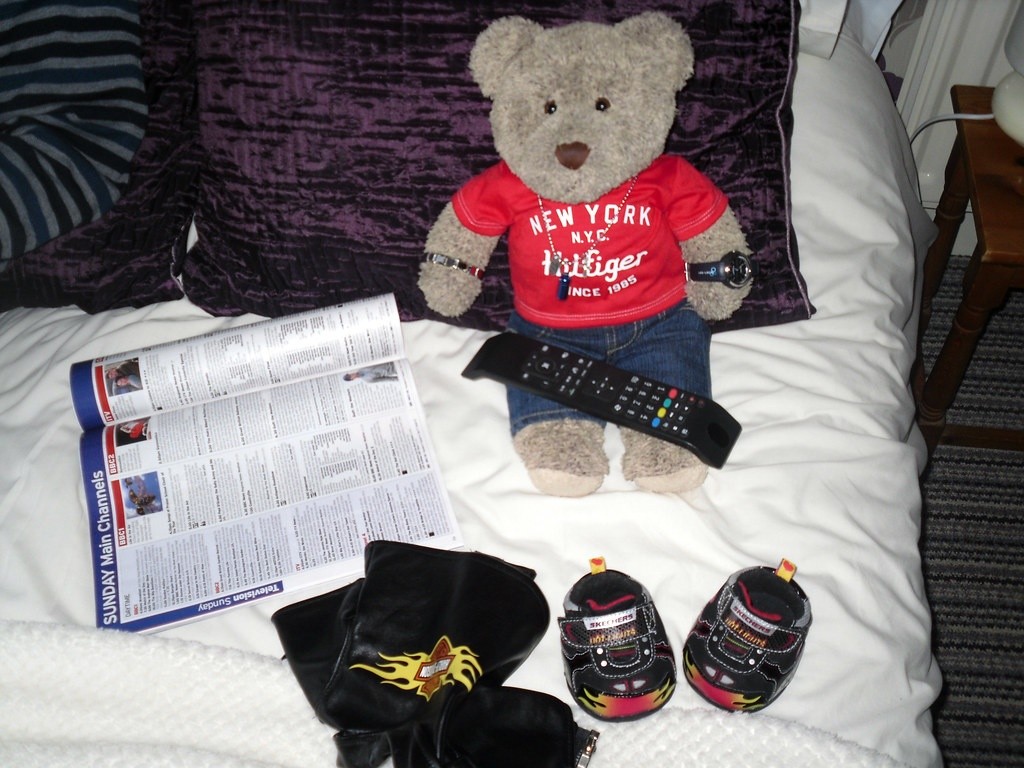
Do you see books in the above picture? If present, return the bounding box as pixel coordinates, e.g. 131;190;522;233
69;289;464;638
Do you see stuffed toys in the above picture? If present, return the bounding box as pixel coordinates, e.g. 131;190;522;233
418;8;758;496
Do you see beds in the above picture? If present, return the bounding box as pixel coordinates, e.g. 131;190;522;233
0;0;950;768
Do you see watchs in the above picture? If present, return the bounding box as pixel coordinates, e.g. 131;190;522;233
682;250;758;288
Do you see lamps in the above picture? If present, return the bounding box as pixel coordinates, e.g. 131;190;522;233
991;0;1024;149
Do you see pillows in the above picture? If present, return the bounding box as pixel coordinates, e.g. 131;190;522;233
0;0;197;316
182;0;814;338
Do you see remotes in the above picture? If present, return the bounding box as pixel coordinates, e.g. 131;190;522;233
462;332;742;470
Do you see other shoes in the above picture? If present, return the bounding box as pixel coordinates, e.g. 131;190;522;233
682;557;812;712
557;555;676;722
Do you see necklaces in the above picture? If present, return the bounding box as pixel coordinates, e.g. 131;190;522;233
537;174;639;302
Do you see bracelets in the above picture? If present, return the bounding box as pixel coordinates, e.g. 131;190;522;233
419;250;485;281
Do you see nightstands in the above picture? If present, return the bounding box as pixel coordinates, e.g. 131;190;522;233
915;80;1024;441
